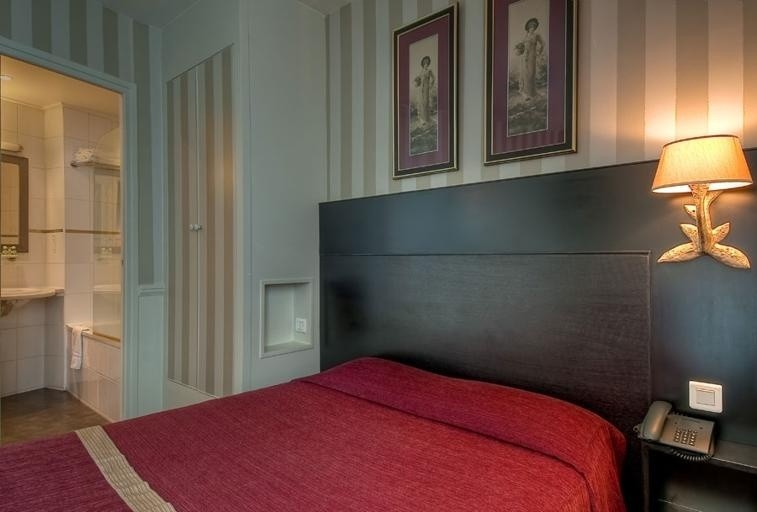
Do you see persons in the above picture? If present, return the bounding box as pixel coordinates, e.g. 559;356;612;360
513;18;545;103
410;54;435;128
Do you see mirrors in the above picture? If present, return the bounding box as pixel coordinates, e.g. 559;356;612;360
0;153;28;254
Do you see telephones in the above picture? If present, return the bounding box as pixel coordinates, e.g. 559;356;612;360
633;401;715;461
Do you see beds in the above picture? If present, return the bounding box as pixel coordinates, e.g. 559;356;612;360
0;146;757;512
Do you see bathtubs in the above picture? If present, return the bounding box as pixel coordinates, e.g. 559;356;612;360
65;320;122;346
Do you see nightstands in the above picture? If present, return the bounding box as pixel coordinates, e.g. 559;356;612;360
641;441;757;512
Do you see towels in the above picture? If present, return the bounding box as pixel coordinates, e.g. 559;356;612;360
70;327;90;368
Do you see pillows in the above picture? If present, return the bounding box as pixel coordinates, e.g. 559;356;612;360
317;357;604;465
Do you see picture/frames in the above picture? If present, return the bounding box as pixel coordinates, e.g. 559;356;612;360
482;0;577;166
391;3;458;180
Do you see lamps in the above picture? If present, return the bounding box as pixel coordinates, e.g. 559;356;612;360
651;133;753;269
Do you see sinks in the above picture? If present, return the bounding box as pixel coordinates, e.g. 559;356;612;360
0;287;64;300
